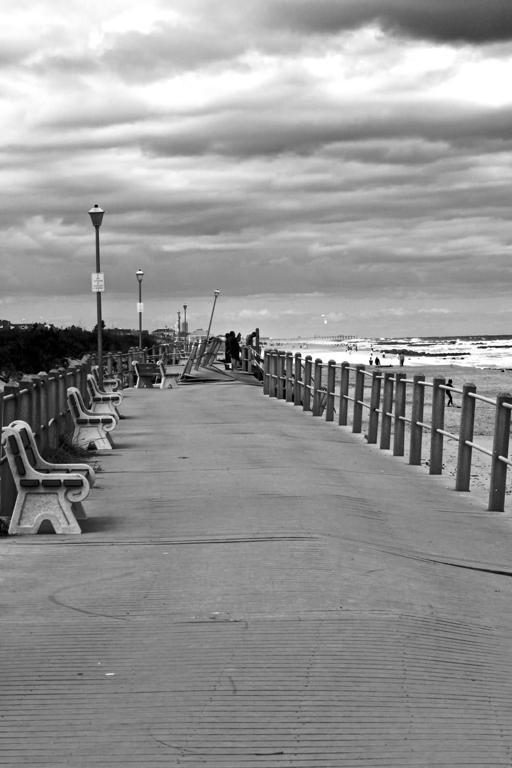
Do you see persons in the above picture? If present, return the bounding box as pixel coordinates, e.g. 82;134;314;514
368;347;404;366
446;379;453;406
347;344;357;355
225;331;256;370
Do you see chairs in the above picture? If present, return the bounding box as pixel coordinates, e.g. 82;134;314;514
2;418;97;535
67;387;116;450
86;373;122;420
90;365;121;392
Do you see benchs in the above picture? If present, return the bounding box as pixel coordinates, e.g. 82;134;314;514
132;361;180;389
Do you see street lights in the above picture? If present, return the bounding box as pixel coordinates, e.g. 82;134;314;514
206;289;221;341
136;269;145;348
88;204;105;392
183;303;188;342
177;310;181;342
